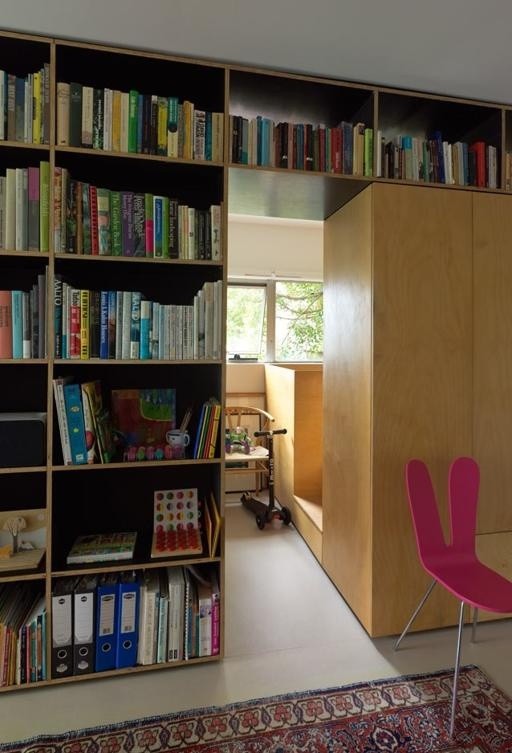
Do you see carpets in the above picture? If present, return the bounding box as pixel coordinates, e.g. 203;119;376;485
0;666;510;752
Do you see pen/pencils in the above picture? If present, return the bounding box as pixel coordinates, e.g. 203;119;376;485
180;408;193;433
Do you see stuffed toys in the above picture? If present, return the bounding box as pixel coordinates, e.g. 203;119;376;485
224;426;257;455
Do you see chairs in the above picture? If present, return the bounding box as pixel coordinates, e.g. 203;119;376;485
224;406;276;504
399;457;512;735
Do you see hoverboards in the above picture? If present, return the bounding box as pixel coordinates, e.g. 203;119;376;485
240;428;293;530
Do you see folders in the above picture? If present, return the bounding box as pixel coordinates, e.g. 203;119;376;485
51;570;141;679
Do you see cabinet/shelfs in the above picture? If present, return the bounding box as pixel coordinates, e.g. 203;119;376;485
226;63;508;223
322;181;509;642
0;23;229;693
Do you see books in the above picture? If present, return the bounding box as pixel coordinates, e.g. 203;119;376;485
1;265;221;361
56;82;224;162
53;377;220;465
1;65;50;144
52;167;221;261
1;161;49;251
2;532;220;687
229;114;511;191
